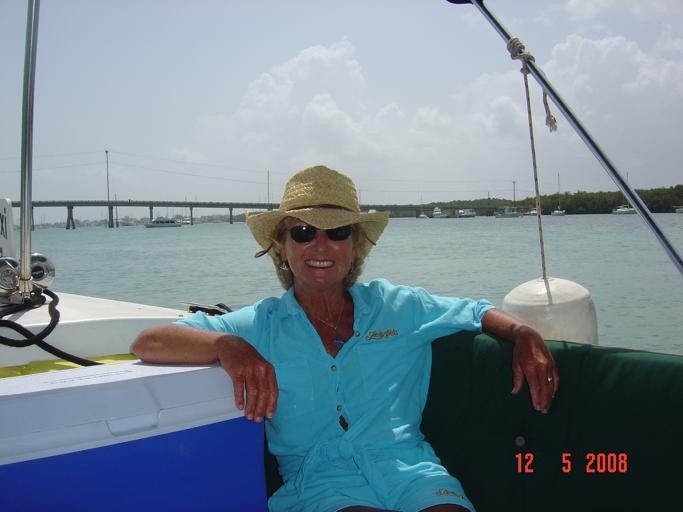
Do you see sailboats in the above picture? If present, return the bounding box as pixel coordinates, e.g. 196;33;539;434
550;172;565;217
611;171;639;216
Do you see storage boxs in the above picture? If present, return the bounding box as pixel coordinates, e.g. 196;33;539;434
0;361;269;512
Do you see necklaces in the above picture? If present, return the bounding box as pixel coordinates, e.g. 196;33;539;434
299;294;347;347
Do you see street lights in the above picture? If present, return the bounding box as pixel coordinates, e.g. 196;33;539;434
143;216;181;228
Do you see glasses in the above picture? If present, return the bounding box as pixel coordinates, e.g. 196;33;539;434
278;222;355;243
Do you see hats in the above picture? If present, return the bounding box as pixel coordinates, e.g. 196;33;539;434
243;164;389;268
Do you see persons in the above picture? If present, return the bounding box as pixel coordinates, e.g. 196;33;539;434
128;162;560;512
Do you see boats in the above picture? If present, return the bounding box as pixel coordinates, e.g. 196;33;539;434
0;1;683;512
419;206;536;219
672;206;683;213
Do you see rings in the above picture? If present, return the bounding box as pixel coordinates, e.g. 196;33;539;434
547;376;554;385
